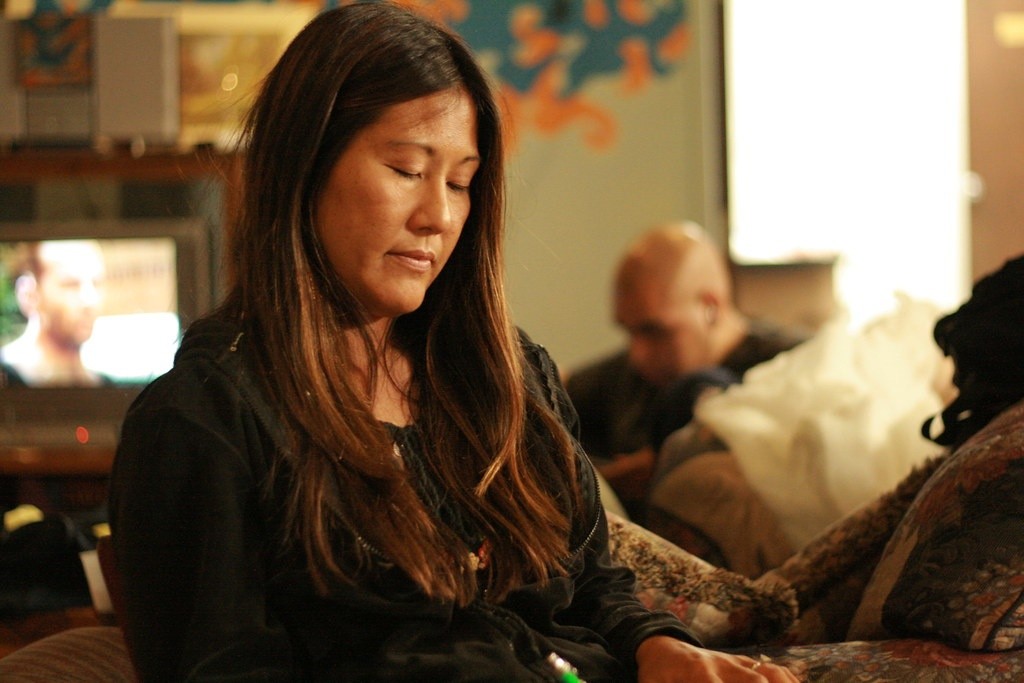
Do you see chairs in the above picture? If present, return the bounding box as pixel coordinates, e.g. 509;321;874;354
0;625;137;683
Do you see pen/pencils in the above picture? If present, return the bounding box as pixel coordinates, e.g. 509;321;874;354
547;651;584;683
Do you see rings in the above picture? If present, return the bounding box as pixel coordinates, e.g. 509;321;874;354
751;653;772;671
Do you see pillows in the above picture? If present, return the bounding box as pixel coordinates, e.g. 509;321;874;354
604;512;799;650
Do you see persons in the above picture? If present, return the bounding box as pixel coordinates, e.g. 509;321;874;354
1;239;116;389
107;0;802;683
560;219;806;529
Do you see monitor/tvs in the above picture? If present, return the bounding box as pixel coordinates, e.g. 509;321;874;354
0;214;210;450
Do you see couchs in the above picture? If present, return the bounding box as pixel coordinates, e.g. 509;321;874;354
650;398;1024;683
